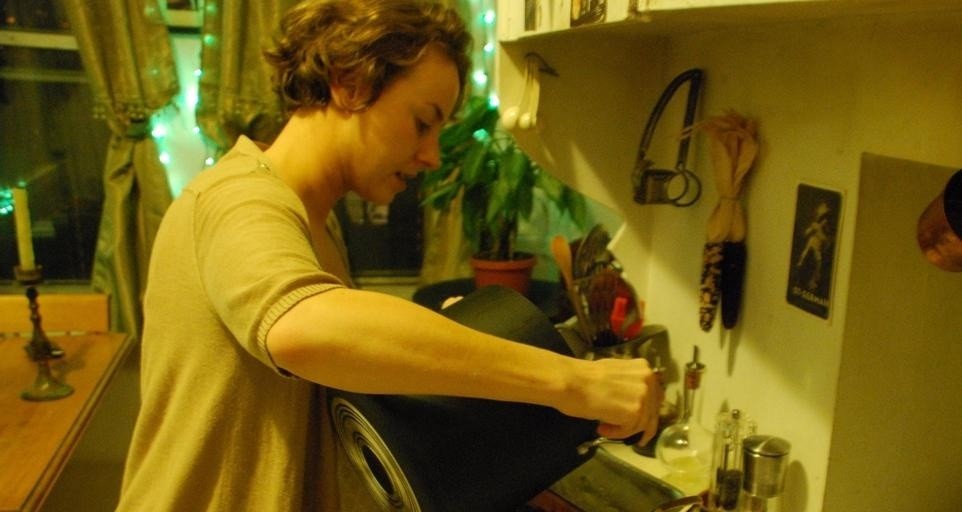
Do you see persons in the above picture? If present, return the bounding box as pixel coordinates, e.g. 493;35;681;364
796;213;831;294
110;2;662;511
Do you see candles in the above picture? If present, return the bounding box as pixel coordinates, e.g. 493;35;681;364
12;188;43;268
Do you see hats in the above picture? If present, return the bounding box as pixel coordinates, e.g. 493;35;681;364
917;171;961;272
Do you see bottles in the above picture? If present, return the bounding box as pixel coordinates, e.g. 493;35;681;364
707;409;791;512
656;362;717;483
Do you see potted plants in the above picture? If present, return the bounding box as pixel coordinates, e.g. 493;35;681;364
416;97;598;295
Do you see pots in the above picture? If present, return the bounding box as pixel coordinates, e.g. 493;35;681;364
328;284;646;512
